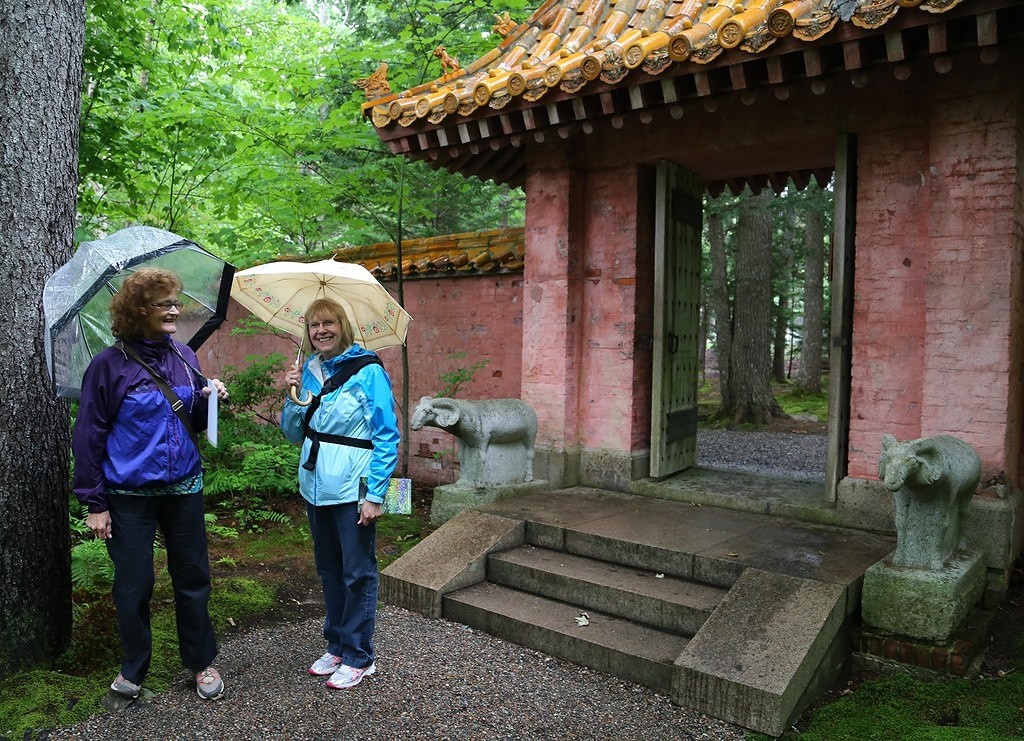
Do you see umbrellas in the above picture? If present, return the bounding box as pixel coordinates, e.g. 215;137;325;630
43;227;237;407
219;259;413;406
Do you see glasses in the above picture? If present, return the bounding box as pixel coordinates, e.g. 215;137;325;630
307;319;339;328
150;300;184;311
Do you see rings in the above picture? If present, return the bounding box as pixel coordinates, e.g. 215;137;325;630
290;376;292;379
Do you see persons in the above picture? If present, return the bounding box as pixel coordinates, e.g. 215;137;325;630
72;267;230;700
281;298;400;689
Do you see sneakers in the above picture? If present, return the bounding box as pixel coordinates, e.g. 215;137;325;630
111;673;142;697
308;652;343;676
187;666;224;700
326;662;376;690
